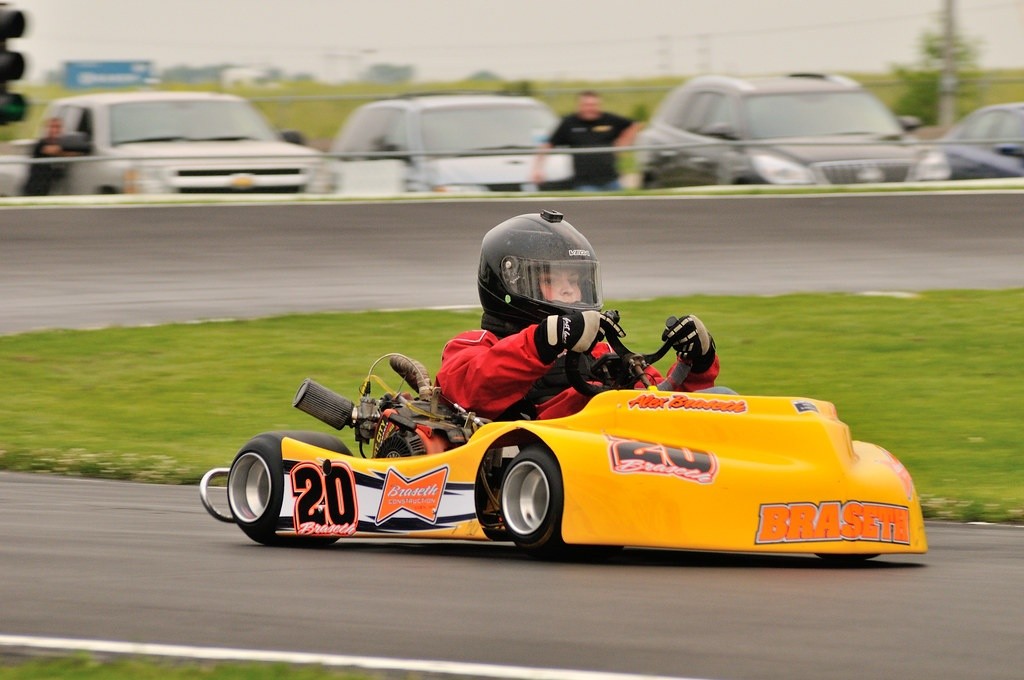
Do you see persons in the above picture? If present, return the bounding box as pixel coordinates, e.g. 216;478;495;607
533;91;635;193
23;117;69;197
434;209;720;422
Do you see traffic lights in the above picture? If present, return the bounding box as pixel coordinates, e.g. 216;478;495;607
0;2;31;127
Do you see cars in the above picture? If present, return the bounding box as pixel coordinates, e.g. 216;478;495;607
934;100;1023;183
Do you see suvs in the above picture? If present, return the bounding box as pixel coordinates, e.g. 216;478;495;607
326;87;579;198
0;92;356;198
632;68;953;190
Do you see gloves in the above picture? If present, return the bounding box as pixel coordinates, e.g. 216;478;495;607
534;310;627;365
661;314;716;373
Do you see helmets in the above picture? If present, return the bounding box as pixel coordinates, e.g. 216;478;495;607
477;209;604;336
